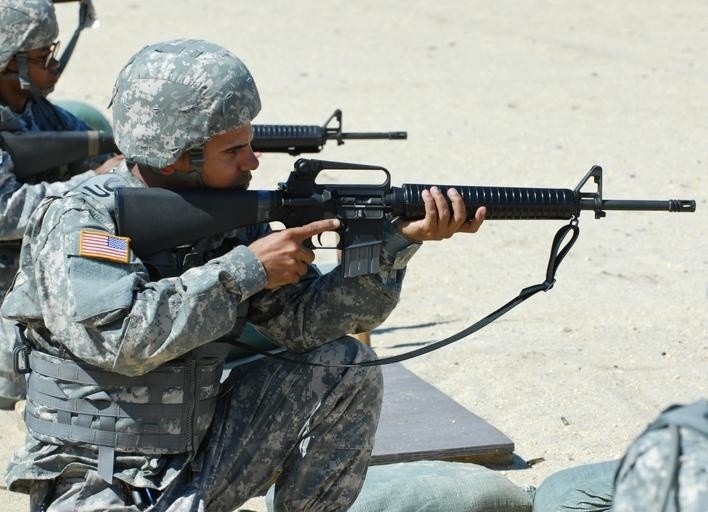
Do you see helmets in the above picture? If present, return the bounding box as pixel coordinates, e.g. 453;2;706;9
107;35;263;170
1;0;60;74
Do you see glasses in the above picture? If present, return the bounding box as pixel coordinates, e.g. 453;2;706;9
16;40;60;69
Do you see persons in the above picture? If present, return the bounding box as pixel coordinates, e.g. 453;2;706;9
0;38;489;512
0;0;123;409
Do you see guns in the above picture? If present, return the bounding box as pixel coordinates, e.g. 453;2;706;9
114;158;695;285
0;110;407;184
